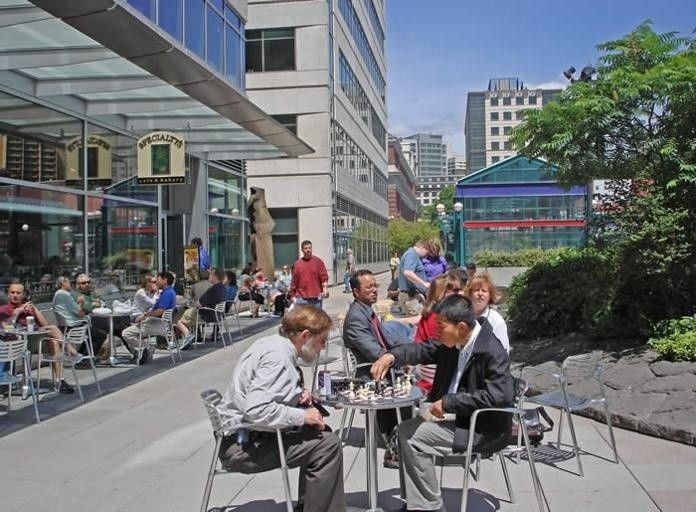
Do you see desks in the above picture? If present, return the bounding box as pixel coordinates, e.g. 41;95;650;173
309;386;424;511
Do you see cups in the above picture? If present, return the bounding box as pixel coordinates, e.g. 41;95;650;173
44;274;51;281
25;316;35;333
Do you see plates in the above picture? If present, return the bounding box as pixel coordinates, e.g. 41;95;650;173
91;308;111;314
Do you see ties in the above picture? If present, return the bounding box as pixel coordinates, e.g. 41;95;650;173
296;365;304;388
371;311;387;348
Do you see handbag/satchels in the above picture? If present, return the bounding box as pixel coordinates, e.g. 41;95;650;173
511;406;553;444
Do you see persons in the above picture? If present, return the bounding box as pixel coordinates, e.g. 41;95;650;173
212;266;514;511
1;272;176;394
175;237;292;350
387;237;477;316
287;238;329;310
343;248;357;293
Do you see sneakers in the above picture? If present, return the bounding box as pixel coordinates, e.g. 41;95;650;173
384;447;402;468
55;332;194;393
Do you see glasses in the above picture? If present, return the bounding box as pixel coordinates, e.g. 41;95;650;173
81;281;90;284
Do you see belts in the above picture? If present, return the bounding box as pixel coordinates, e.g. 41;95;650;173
400;290;419;294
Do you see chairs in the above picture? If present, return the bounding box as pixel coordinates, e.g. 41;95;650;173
432;375;553;512
338;313;416;441
0;282;184;425
200;388;294;512
184;272;289;349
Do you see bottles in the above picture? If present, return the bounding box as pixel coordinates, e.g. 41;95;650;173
183;287;192;306
384;306;394;320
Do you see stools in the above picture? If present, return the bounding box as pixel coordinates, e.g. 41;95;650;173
515;365;619;479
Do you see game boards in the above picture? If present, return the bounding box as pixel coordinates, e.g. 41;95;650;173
331;376;408;404
